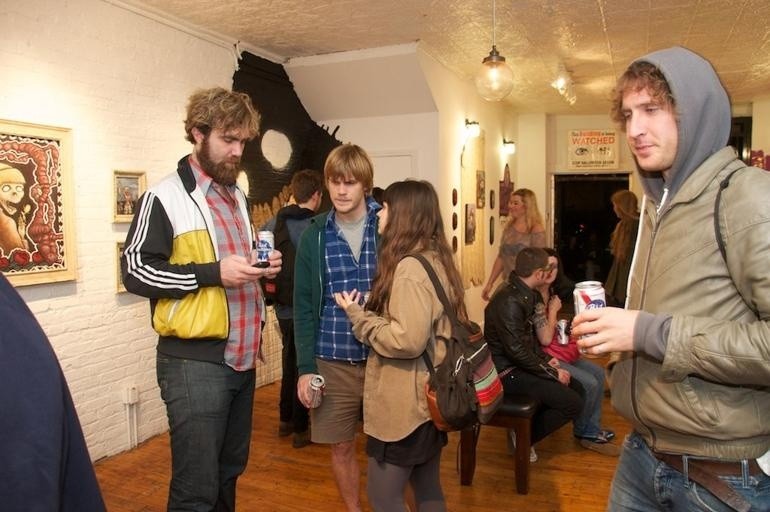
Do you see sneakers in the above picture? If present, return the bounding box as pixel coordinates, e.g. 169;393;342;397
294;430;312;447
279;422;296;436
511;430;537;462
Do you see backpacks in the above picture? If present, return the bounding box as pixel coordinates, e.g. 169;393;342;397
402;252;503;431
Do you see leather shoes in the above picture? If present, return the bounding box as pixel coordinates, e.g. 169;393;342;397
574;429;614;443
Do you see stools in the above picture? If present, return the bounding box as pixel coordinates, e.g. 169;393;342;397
462;392;545;493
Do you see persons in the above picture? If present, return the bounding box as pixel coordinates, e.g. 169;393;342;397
536;248;623;458
333;180;468;511
118;185;134;214
484;246;585;462
261;170;326;446
0;273;107;511
293;144;384;511
569;46;770;511
605;189;642;307
481;188;548;301
122;87;282;511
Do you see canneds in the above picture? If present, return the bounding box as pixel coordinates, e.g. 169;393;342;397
306;374;327;409
572;281;608;360
556;318;569;346
256;230;275;266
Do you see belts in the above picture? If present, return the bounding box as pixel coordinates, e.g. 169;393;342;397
655;452;762;511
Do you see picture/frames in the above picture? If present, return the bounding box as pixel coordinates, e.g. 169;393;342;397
1;118;79;292
113;173;145;295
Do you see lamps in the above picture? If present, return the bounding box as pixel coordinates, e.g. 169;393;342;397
478;0;515;101
